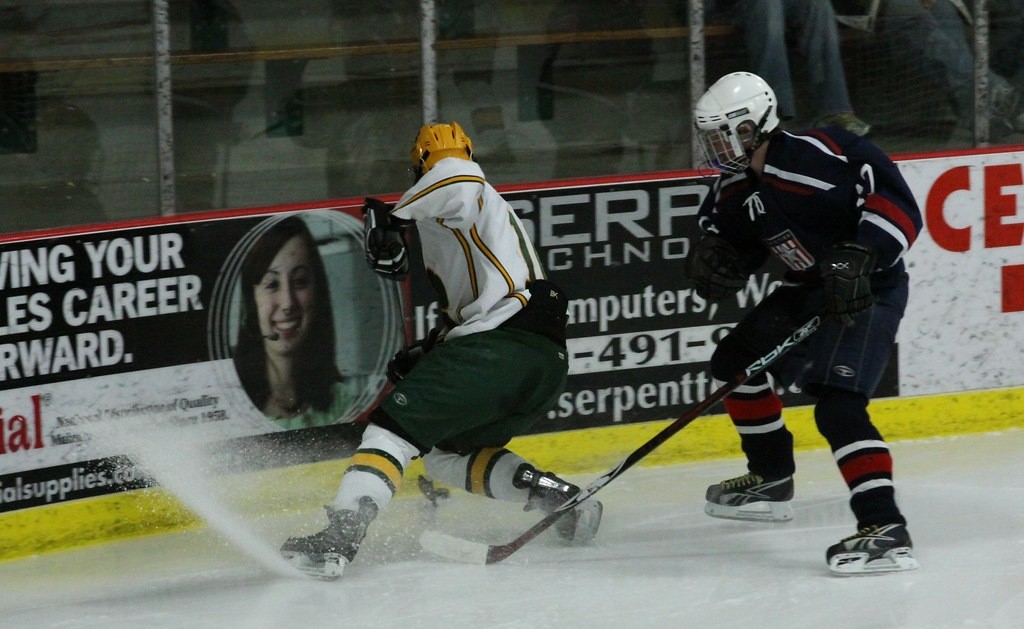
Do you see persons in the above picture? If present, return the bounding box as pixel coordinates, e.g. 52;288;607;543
278;120;604;579
685;71;920;574
832;0;1024;138
235;216;364;428
673;0;870;135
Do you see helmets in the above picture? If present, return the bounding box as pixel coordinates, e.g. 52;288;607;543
411;121;473;173
695;71;780;134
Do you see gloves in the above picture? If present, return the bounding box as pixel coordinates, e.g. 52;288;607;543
385;327;440;385
688;234;735;303
361;203;410;281
819;246;878;328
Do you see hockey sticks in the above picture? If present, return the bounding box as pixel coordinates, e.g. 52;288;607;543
417;304;836;567
369;209;452;502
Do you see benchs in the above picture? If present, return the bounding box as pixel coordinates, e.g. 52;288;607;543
0;19;974;157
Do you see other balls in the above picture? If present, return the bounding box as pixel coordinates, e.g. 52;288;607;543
433;488;449;506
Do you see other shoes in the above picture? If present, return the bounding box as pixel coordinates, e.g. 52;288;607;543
816;113;873;137
988;82;1024;142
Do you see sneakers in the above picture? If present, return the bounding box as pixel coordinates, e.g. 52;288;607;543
826;525;919;574
706;473;796;521
513;463;603;544
281;496;378;578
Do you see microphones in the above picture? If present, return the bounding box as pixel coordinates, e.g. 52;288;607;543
254;333;279;341
